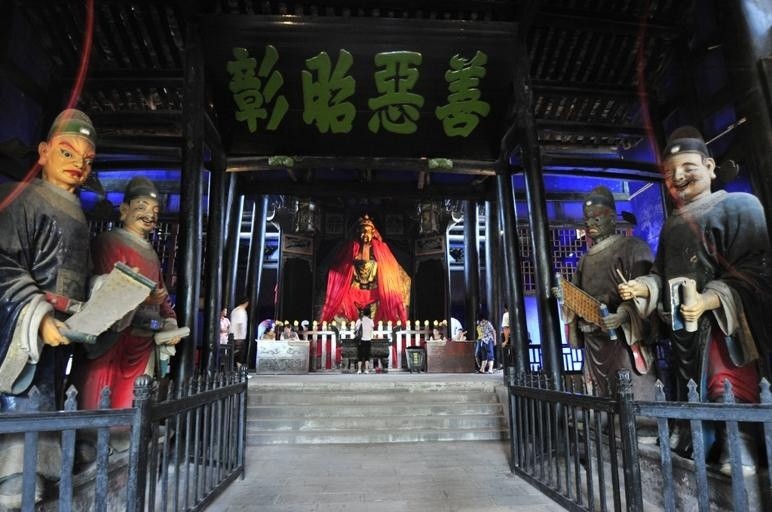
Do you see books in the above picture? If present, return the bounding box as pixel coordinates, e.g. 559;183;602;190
154;327;191;346
668;276;698;333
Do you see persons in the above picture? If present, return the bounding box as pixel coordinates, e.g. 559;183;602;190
261;326;276;340
280;324;298;341
617;125;772;475
0;108;92;510
321;215;411;329
230;296;252;377
428;304;511;373
353;308;375;373
551;185;670;445
218;308;230;345
67;175;181;464
